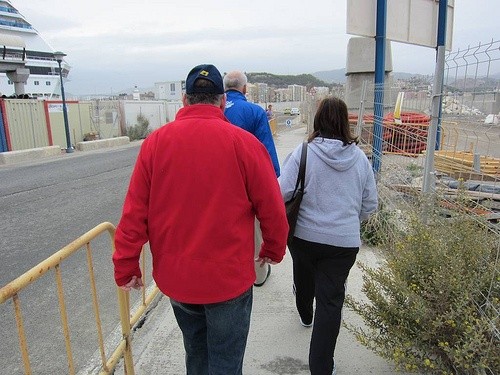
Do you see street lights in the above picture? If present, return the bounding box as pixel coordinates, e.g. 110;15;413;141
53;52;75;153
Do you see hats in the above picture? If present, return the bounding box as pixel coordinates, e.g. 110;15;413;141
185;64;225;95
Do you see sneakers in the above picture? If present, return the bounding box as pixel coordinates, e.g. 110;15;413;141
298;310;313;328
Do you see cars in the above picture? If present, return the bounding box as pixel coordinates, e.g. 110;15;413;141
284;107;291;114
290;108;300;115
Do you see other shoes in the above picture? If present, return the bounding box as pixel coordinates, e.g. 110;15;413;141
254;264;272;287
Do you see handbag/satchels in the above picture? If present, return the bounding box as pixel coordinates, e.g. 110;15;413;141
284;190;305;246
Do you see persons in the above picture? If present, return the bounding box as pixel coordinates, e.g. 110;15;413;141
197;70;282;287
111;64;291;375
281;96;378;375
267;105;274;119
